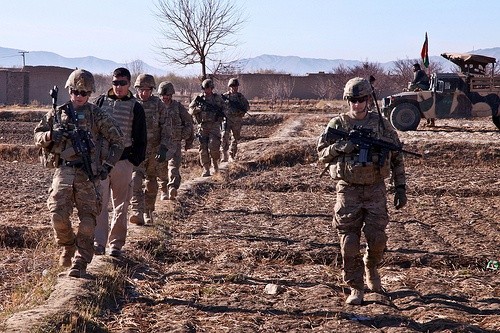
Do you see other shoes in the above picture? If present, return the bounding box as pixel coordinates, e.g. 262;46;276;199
425;121;436;126
109;246;120;255
93;244;106;255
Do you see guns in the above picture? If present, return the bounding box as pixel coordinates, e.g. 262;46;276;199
319;125;423;180
221;93;253;118
195;96;234;124
59;103;102;201
49;84;59;108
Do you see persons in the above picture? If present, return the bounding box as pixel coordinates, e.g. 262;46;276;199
424;118;435;128
315;77;408;306
187;78;228;176
155;81;193;201
221;78;250;161
407;64;430;91
83;66;147;258
33;67;124;278
127;73;169;225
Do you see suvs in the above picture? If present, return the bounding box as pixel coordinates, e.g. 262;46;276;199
381;51;500;133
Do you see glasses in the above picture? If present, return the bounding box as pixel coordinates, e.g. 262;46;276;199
112;79;129;86
71;89;90;96
347;95;368;102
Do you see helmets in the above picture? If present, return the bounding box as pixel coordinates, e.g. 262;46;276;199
135;73;156;88
64;69;96;92
202;78;214;87
228;77;240;86
343;76;373;97
157;81;175;94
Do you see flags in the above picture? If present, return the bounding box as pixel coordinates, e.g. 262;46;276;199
420;33;430;67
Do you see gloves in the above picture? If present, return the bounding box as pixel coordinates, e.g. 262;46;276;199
333;140;355;153
393;188;408;208
51;129;69;142
98;163;113;179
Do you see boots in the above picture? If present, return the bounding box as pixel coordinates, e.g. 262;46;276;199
168;186;178;199
211;161;218;174
230;151;236;160
202;162;210;176
68;256;87;276
143;213;154;225
365;263;381;290
221;152;229;161
160;187;169;199
346;280;365;304
130;211;145;226
58;244;75;266
